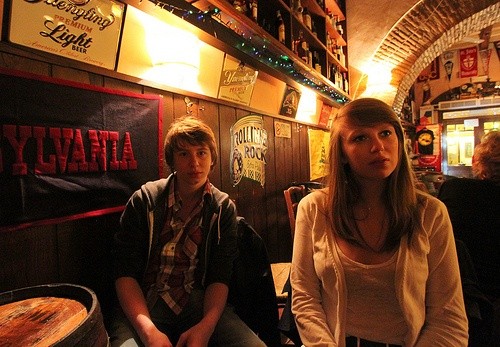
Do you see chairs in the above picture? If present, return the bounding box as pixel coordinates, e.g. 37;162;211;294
228;216;282;347
284;184;307;242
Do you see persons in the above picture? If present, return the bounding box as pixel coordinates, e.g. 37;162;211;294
289;98;469;346
112;115;268;347
438;131;500;347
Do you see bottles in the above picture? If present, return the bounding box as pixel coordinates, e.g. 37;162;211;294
226;0;349;94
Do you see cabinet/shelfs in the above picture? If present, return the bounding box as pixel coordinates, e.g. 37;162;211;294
212;0;350;95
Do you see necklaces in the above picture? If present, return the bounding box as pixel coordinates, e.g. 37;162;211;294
365;196;385;245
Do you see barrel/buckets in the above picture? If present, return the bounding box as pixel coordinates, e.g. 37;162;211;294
0;284;110;347
